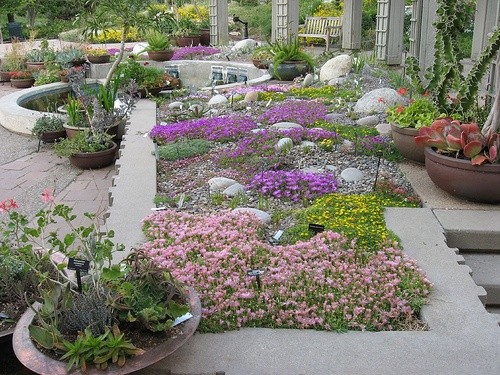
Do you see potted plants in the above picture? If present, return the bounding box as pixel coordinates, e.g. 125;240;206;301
413;115;500;204
0;17;316;170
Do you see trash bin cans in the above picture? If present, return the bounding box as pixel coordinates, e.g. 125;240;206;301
7;13;14;23
6;22;23;42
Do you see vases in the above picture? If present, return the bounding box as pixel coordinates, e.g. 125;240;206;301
175;33;202;47
0;249;77;351
10;79;35;88
389;120;425;166
123;81;171;99
87;54;110;64
13;269;203;375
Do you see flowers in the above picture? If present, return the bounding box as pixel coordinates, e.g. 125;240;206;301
174;26;192;38
8;70;31;79
0;186;192;375
110;54;171;93
86;46;108;56
377;87;447;130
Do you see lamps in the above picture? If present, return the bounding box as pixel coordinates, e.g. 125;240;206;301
306;223;325;237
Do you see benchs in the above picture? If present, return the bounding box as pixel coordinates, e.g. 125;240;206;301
298;13;344;52
